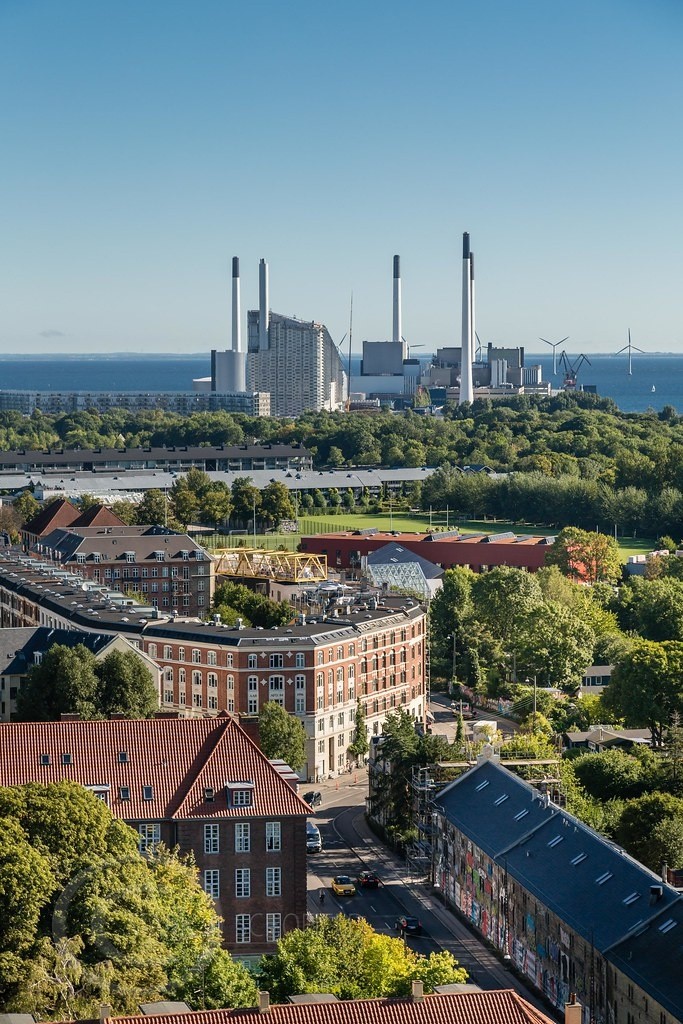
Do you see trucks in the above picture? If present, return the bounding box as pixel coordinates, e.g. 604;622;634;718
307;821;324;853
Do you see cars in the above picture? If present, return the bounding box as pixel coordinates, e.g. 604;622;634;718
395;915;422;937
356;871;379;888
451;702;473;719
331;876;356;896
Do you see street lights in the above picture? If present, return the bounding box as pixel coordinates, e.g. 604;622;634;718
295;479;298;533
447;633;456;677
451;699;462;722
525;675;536;737
251;493;257;548
165;483;168;526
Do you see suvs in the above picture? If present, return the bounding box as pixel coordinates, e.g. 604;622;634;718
302;790;322;809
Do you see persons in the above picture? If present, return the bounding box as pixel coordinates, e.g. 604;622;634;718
319;889;325;898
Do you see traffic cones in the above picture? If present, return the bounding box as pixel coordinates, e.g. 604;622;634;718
353;774;358;783
336;780;339;788
